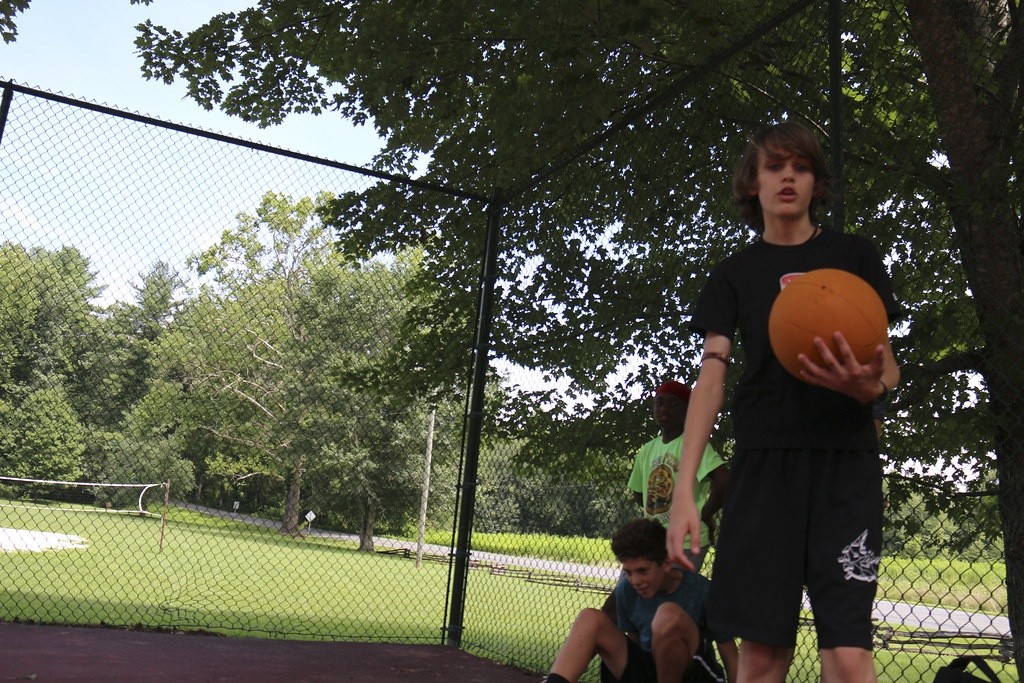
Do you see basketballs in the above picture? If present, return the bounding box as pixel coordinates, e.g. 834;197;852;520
770;268;890;380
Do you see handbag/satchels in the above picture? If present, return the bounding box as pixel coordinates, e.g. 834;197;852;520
933;655;1000;683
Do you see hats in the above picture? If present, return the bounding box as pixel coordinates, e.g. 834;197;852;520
655;382;691;403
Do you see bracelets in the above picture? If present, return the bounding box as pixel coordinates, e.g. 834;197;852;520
699;351;732;367
880;380;890;402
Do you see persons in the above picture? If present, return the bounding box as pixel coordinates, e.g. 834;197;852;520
668;120;909;682
538;517;739;683
626;377;730;573
